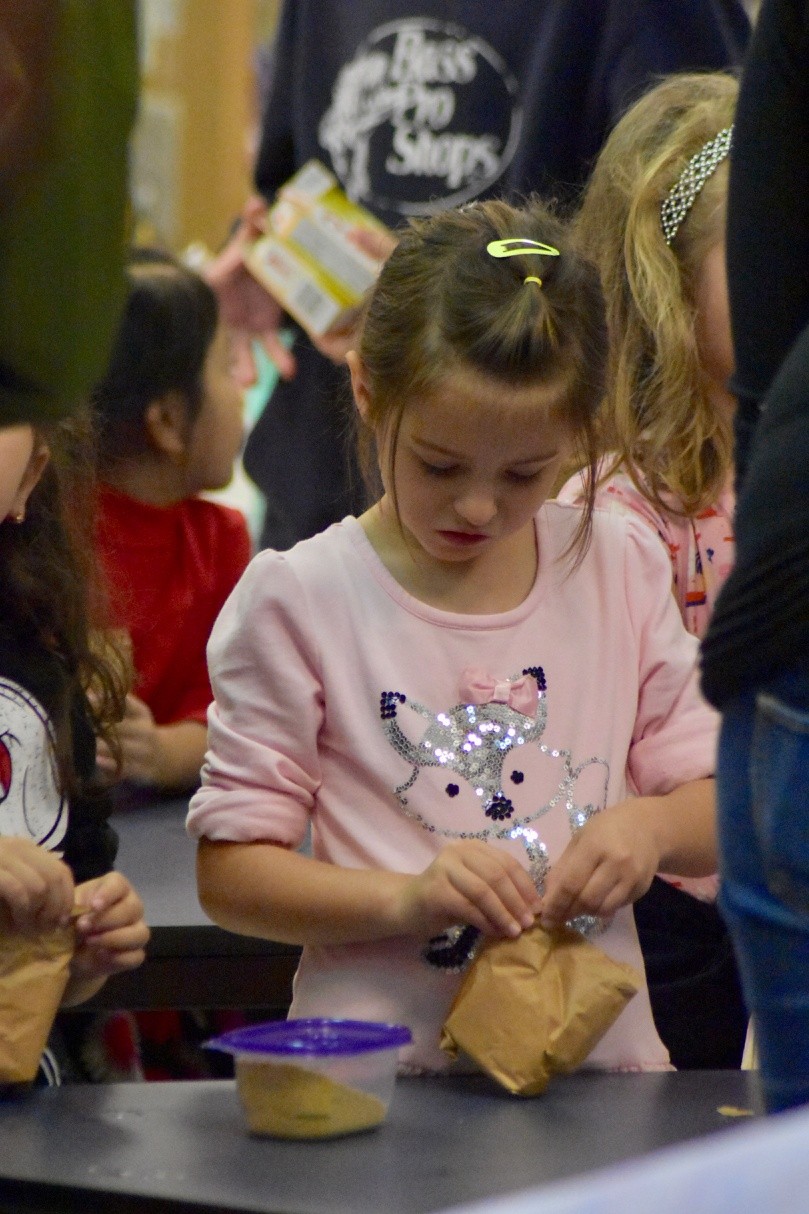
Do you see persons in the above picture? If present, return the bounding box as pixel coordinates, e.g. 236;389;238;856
75;247;251;792
0;0;149;1090
699;0;809;1069
556;70;750;1070
185;199;725;1071
203;0;751;555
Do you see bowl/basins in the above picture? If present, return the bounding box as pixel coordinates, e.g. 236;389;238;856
206;1019;412;1141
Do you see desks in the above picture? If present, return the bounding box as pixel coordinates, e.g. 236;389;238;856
0;1069;809;1214
51;784;312;1080
